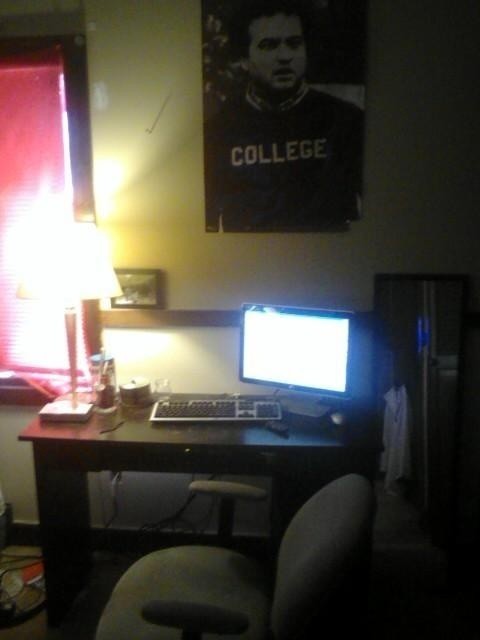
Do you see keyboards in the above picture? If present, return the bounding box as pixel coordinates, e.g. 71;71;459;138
148;399;282;422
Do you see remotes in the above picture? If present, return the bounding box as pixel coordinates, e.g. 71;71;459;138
265;421;290;433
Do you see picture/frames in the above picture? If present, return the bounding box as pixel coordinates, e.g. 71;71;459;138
111;268;165;307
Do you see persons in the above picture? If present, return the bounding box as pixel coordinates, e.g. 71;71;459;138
202;1;366;235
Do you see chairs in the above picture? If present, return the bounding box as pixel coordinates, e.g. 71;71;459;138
95;473;378;639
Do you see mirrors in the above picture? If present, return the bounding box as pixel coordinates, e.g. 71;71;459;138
376;275;471;548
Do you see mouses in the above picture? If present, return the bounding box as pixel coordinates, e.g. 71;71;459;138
330;410;344;425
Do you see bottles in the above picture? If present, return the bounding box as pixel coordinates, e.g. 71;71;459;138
90;355;120;409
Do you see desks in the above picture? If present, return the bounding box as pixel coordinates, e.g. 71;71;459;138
18;392;379;628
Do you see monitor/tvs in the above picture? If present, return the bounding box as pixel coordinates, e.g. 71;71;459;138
238;302;356;418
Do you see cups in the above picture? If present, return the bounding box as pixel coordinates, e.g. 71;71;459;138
155;378;171;402
